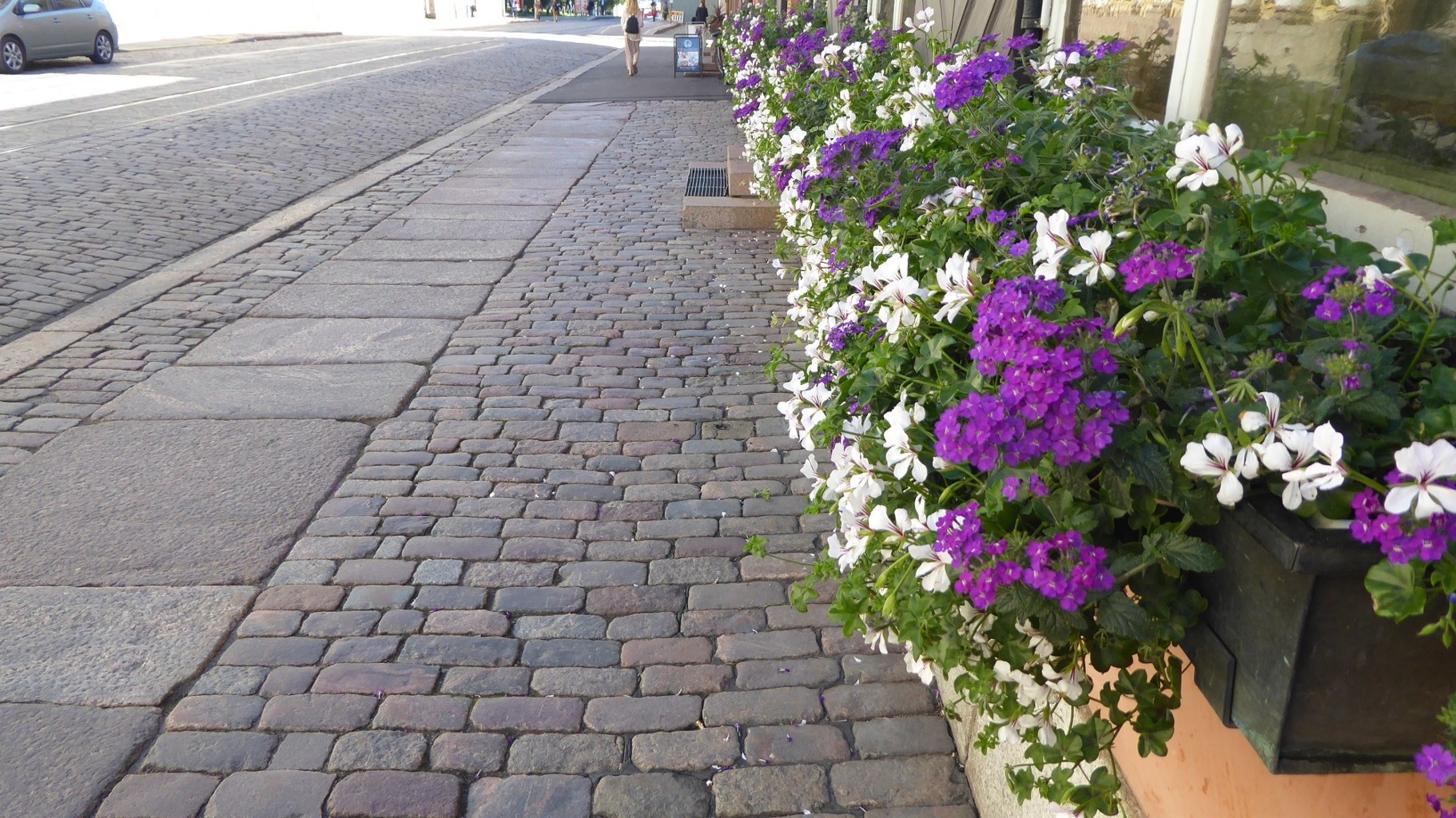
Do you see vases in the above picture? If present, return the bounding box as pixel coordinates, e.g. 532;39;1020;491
1183;491;1456;777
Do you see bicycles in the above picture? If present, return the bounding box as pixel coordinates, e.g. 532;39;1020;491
709;13;728;78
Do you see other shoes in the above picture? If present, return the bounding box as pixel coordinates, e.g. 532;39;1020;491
629;64;638;76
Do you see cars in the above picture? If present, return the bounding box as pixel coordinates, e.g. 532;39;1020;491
0;0;120;76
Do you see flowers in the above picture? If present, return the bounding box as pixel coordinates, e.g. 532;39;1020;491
713;0;1456;818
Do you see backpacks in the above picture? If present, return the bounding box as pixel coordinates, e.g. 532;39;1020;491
625;13;639;34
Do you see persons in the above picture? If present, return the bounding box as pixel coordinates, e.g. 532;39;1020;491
650;0;669;22
695;0;708;27
620;0;644;76
510;0;607;23
